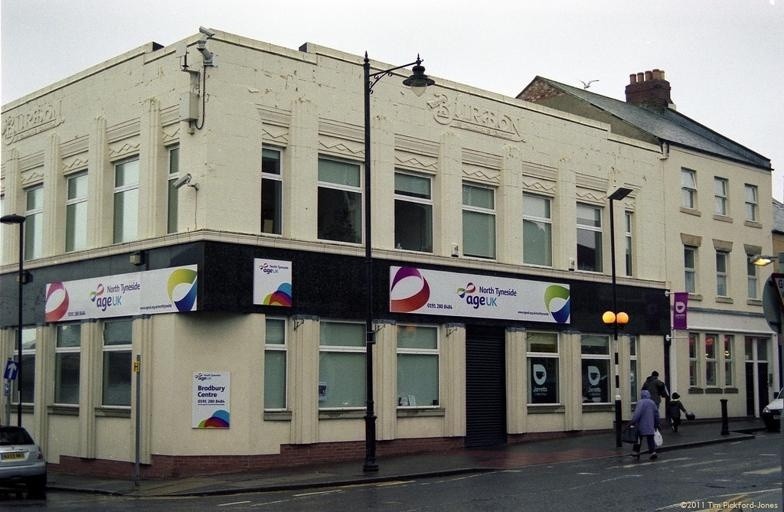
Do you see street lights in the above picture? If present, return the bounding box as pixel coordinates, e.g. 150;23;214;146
608;186;636;447
358;50;437;470
753;250;783;272
0;211;25;427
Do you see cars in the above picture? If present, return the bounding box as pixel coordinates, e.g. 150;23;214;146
761;386;784;431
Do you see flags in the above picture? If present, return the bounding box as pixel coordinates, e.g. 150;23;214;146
672;291;687;330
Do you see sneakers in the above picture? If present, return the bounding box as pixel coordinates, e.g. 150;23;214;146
629;453;640;461
649;453;658;460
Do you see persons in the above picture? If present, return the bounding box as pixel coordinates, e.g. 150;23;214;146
640;371;669;430
624;388;660;464
666;391;687;432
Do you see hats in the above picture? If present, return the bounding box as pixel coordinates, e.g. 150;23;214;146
652;371;659;377
672;393;681;399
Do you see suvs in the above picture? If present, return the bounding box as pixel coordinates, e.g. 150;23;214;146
0;424;47;497
